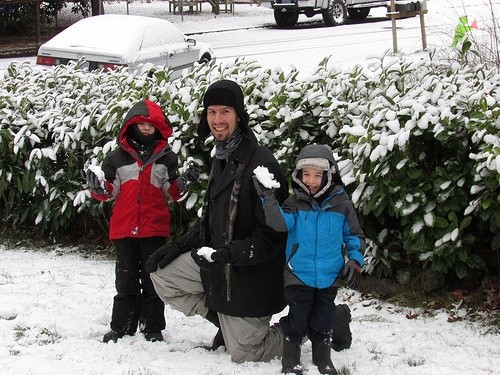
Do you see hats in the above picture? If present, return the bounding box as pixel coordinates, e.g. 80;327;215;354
197;80;249;137
292;157;336;200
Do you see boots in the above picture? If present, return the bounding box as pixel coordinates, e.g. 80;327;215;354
312;344;338;375
282;338;303;375
332;304;352;351
205;309;227;352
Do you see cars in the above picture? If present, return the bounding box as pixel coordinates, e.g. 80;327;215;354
36;14;216;82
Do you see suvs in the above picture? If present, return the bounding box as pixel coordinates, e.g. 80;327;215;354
270;0;421;26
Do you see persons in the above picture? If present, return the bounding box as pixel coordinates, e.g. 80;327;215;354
252;144;366;375
86;99;200;343
145;80;352;364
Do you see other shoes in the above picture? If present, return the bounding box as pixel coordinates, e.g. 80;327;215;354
103;329;134;343
144;330;164;342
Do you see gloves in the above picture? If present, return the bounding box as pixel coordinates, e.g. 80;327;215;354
145;243;180;272
191;247;230;270
341;259;361;290
80;169;103;195
183;161;200;182
252;175;275;197
